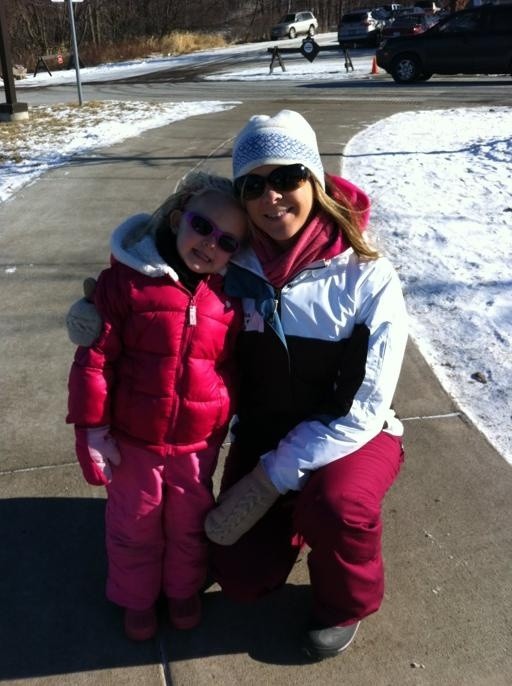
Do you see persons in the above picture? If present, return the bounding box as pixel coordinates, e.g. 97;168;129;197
63;108;410;658
65;171;258;642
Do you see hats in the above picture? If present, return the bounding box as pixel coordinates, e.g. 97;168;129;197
231;109;326;192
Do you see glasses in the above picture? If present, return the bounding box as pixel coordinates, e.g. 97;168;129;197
233;163;312;201
178;203;243;255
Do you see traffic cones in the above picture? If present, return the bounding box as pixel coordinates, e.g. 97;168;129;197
370;56;380;74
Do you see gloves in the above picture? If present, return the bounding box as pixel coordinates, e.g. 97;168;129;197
74;424;122;486
204;460;281;547
65;276;104;348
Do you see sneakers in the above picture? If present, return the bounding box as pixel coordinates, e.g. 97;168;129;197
304;607;361;660
124;603;157;641
167;589;203;631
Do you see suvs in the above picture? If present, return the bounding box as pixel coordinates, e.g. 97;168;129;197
271;9;319;38
376;1;512;83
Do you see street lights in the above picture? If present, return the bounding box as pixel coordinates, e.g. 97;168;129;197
52;0;85;107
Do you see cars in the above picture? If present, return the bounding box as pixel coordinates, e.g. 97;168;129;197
338;2;447;48
